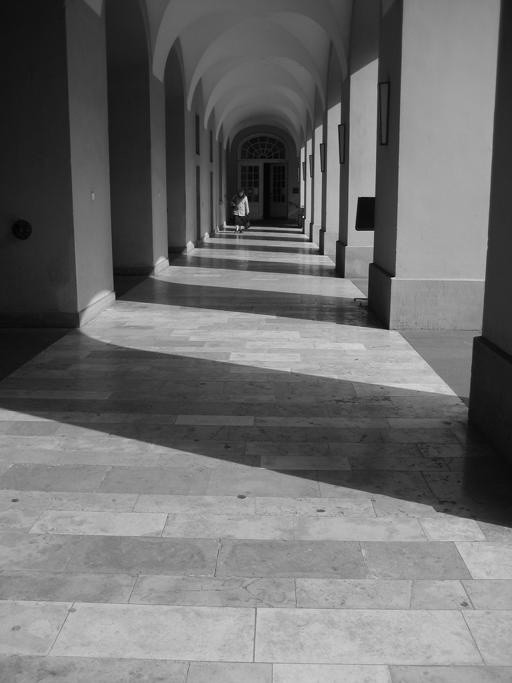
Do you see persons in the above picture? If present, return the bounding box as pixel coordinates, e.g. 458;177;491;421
230;189;250;234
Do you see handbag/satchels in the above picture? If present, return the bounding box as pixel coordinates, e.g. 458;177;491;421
245;222;250;228
